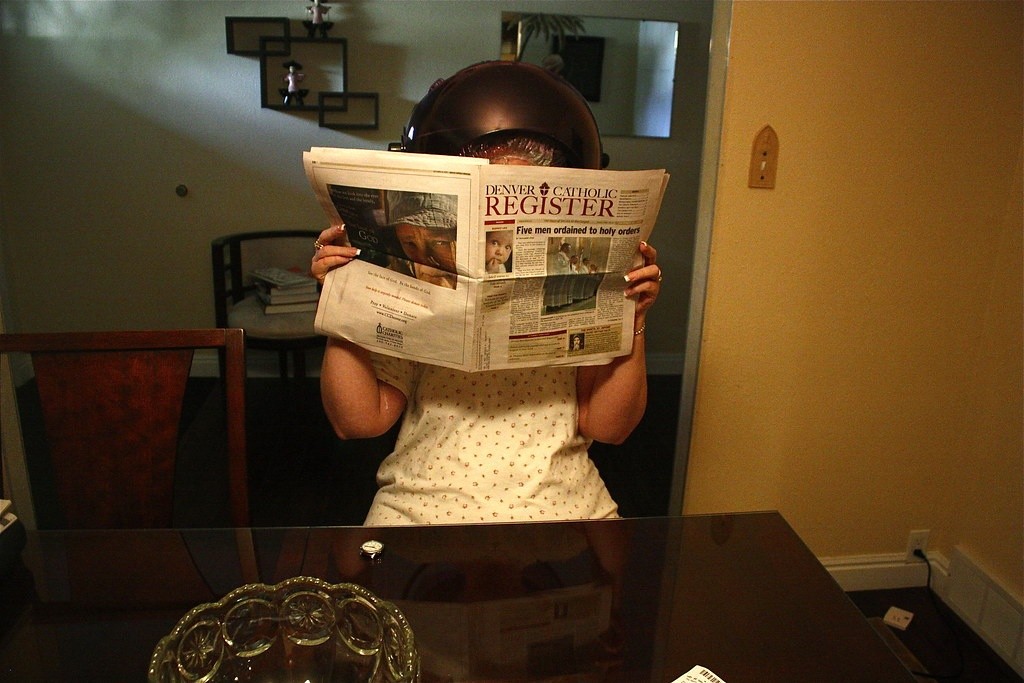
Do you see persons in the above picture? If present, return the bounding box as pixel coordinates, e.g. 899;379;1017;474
311;60;659;526
384;190;458;290
306;0;332;37
485;230;513;274
282;60;305;105
570;335;583;350
544;243;599;312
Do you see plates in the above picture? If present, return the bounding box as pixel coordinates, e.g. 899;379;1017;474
147;576;421;682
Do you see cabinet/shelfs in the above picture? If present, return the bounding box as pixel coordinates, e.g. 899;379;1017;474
225;16;379;130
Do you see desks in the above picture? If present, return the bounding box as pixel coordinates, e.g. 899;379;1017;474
0;509;917;683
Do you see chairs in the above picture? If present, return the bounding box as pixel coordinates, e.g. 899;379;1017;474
211;229;326;413
29;526;261;629
0;327;252;528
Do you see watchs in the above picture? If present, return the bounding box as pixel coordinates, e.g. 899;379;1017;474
359;540;385;560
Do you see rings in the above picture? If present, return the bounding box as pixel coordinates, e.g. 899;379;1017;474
314;238;323;250
656;269;663;281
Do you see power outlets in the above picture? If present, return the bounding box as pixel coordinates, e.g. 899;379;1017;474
905;529;930;563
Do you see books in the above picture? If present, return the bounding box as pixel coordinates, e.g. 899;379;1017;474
248;267;319;316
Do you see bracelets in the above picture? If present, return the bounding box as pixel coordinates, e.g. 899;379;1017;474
634;322;646;335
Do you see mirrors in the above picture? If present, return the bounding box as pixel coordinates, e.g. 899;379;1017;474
499;10;681;139
